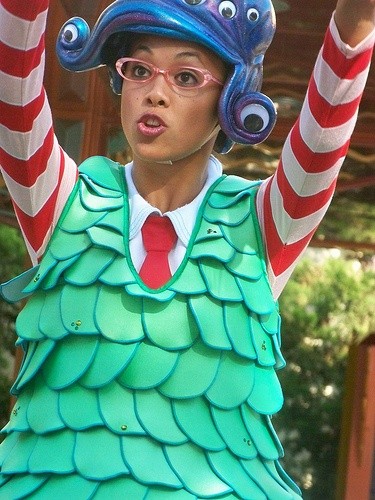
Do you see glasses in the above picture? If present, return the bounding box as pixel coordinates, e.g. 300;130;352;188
114;56;224;93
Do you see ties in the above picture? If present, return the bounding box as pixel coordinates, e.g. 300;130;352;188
137;214;178;290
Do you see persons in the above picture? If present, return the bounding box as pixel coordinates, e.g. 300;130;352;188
1;1;375;500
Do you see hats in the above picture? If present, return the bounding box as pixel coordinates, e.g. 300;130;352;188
56;0;277;155
